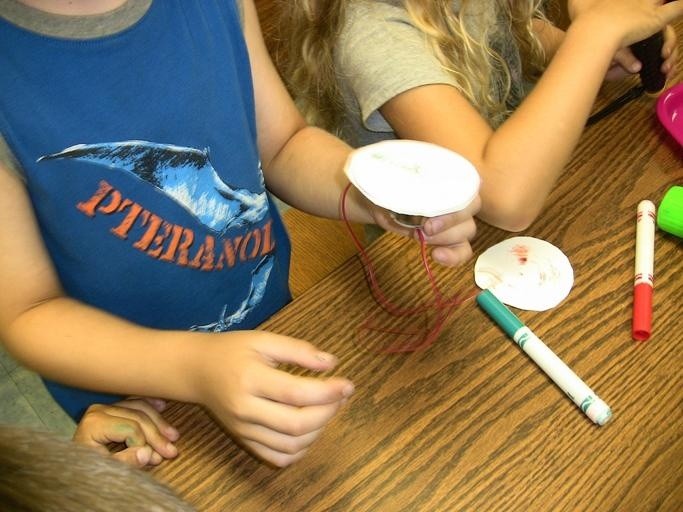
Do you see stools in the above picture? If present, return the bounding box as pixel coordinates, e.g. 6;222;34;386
261;206;368;303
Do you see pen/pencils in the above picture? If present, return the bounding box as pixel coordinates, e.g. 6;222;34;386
475;289;613;426
632;199;655;341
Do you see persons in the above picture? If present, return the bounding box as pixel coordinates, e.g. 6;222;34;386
259;2;682;236
0;392;184;512
1;0;483;470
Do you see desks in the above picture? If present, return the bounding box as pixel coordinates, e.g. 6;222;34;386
107;13;683;512
